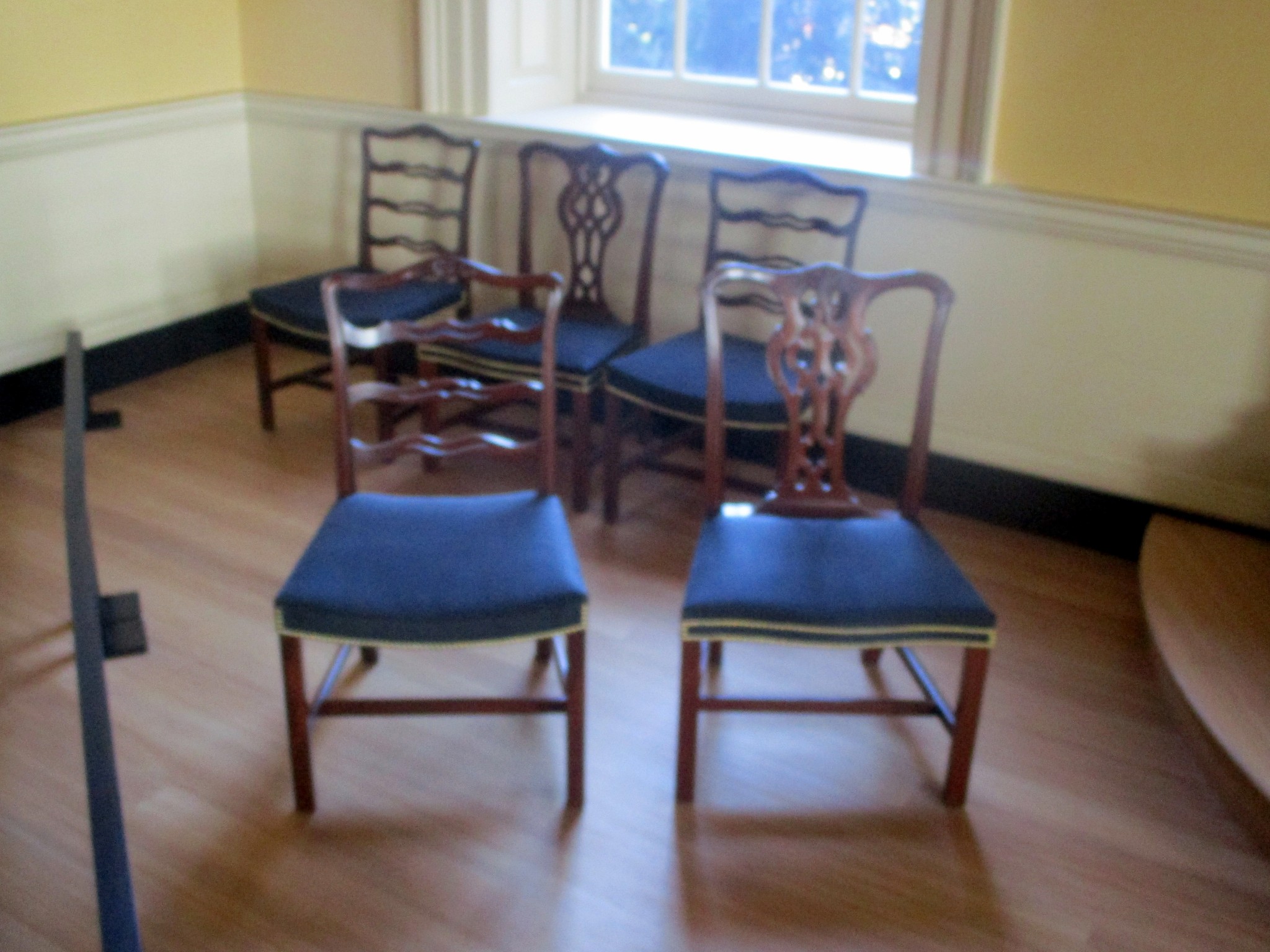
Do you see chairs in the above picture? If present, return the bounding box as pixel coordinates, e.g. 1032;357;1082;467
673;262;999;803
603;162;873;522
244;125;481;463
274;251;593;816
414;144;664;511
